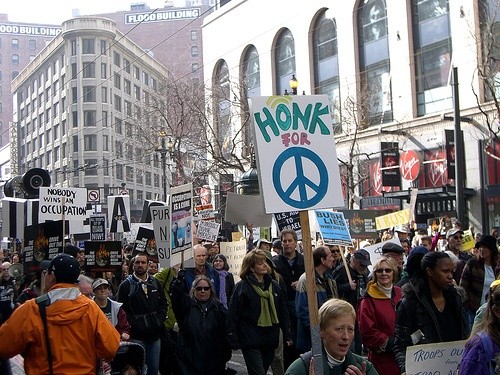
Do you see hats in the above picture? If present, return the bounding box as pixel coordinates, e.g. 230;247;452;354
359;241;370;249
475;234;498;254
354;249;372;265
257;238;272;250
92;280;111;290
446;227;465;240
395;226;411;234
272;240;282;247
49;253;81;281
490;280;500;292
382;243;406;255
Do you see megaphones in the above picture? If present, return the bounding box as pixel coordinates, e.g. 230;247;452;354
8;263;24;285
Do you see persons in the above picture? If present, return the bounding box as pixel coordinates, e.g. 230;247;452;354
0;218;500;375
284;299;379;375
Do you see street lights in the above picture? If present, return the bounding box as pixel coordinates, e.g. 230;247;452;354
154;128;170;203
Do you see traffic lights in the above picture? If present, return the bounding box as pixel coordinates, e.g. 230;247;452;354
22;168;51;196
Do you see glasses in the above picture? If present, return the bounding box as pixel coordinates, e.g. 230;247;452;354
331;250;341;253
195;286;211;291
450;235;463;240
374;267;392;273
2;268;10;271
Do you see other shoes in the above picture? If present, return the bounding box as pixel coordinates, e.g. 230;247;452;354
224;367;237;375
